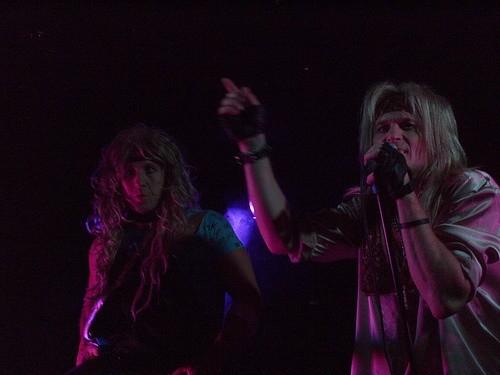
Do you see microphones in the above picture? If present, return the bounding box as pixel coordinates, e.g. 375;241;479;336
361;144;397;176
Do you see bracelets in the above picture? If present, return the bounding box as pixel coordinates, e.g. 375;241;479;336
173;366;196;374
234;146;274;165
398;218;430;229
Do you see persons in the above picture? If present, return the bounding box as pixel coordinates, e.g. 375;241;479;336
218;75;500;375
72;128;260;375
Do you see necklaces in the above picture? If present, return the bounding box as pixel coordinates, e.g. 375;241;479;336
390;219;406;275
